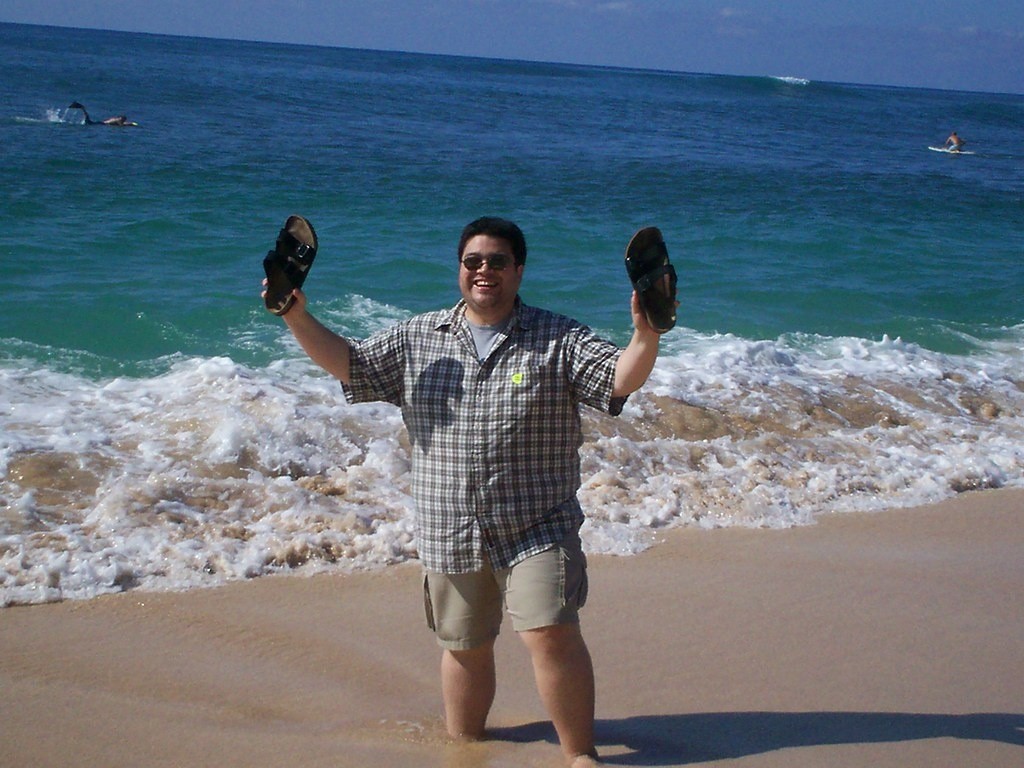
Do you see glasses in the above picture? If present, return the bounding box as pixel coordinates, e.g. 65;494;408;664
460;255;521;271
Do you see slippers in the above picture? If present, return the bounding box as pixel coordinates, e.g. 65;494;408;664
262;214;319;317
624;227;679;334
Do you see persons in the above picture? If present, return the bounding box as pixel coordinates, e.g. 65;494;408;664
945;132;965;152
68;99;131;127
260;216;681;768
